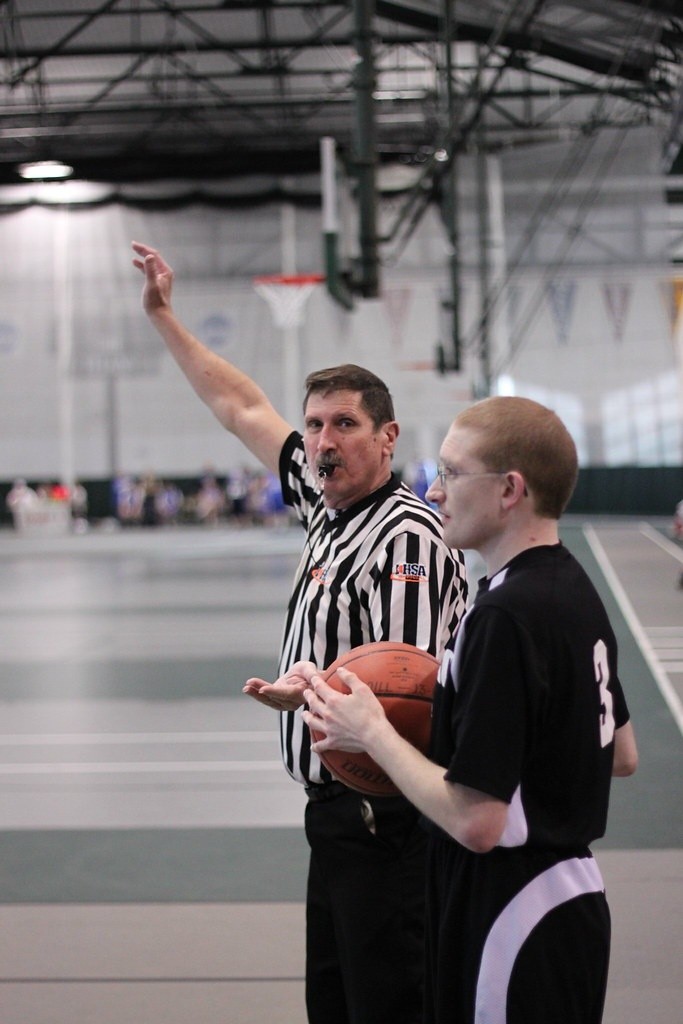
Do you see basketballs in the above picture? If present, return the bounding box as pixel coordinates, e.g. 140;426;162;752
310;642;443;801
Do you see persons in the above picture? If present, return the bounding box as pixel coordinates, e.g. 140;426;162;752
6;454;439;532
126;240;468;1024
300;395;639;1024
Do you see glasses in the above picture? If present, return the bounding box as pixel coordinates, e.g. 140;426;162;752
438;465;529;497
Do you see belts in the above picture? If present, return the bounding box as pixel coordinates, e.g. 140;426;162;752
304;781;349;801
